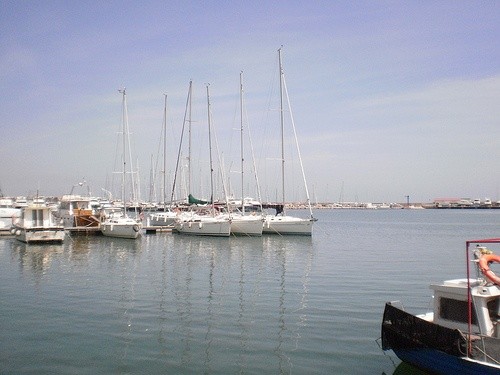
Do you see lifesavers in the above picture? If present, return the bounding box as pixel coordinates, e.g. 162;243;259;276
479;254;500;285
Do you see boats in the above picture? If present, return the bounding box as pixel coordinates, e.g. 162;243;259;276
11;204;66;246
379;237;500;374
60;192;92;233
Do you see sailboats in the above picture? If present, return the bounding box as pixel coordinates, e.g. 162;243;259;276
231;71;263;238
174;83;231;237
99;87;143;238
262;44;317;236
147;81;194;226
